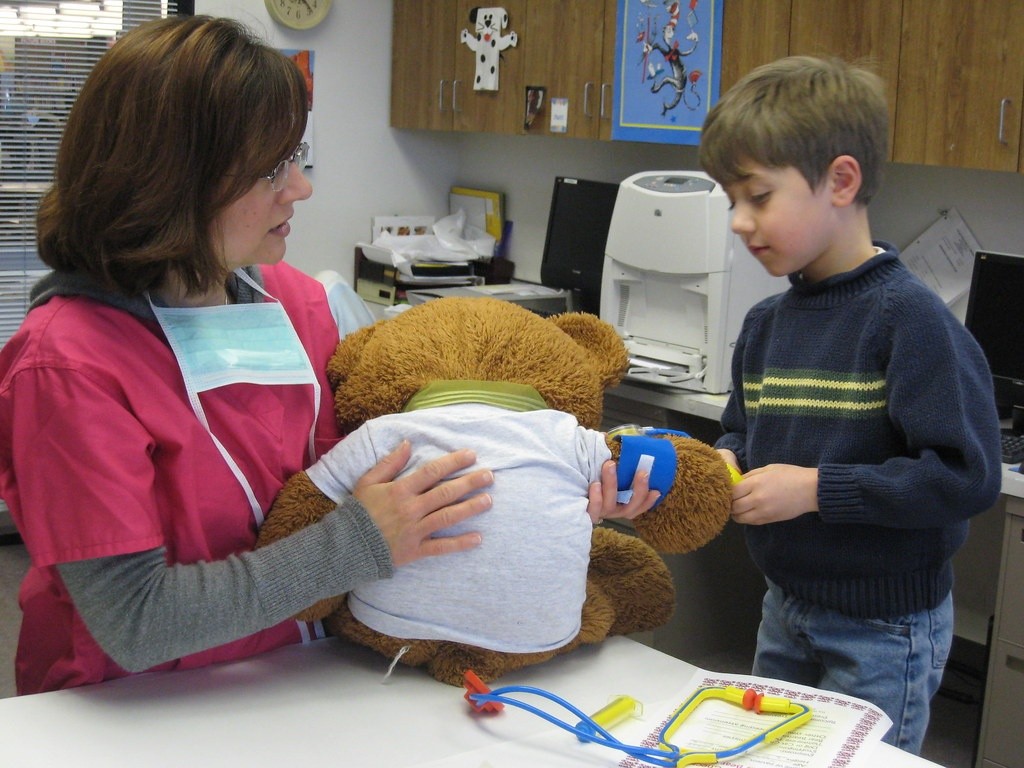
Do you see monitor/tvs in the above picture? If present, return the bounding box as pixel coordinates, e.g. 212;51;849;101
964;249;1024;435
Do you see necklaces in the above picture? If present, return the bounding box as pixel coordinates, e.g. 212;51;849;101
224;294;230;306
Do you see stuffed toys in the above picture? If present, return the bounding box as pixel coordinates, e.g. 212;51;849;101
254;297;733;687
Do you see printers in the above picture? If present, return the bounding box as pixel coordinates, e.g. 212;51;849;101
405;281;572;318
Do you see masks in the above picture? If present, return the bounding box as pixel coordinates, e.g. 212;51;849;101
141;267;325;642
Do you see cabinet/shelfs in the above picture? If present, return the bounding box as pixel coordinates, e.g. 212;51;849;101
581;397;747;658
391;0;1024;169
974;500;1024;768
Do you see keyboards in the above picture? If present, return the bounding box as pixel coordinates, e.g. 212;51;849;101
1001;434;1024;463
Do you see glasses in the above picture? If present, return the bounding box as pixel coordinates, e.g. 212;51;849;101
222;141;310;192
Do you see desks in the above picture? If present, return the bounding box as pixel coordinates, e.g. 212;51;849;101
0;639;951;768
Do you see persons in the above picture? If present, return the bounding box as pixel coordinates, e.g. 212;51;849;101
1;14;661;700
700;51;1001;758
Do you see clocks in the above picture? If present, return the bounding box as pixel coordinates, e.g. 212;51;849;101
265;0;333;29
